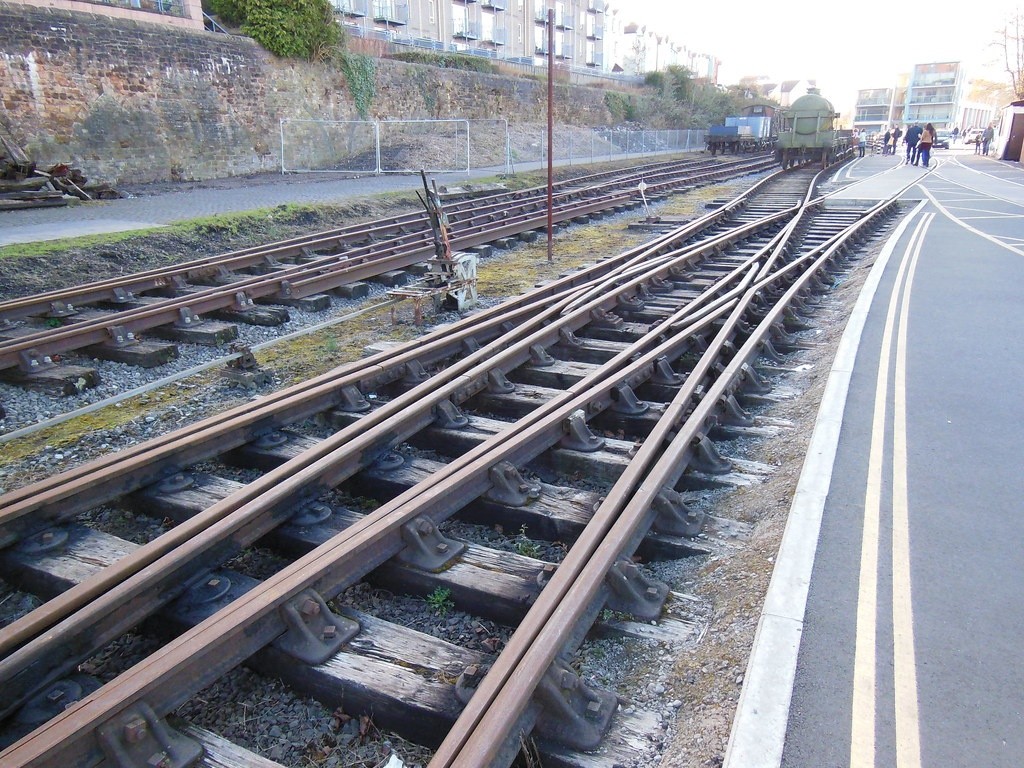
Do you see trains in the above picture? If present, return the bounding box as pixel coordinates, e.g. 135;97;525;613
772;88;855;171
704;104;789;156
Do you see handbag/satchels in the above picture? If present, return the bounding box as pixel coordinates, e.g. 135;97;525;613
982;137;986;142
921;142;931;150
859;142;865;147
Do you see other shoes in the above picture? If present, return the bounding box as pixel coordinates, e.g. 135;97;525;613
913;164;918;166
906;159;910;165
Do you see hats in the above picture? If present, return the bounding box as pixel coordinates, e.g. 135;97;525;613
861;128;865;132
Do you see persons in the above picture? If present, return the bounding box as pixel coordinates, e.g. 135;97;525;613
961;129;967;144
974;122;994;155
851;128;867;157
883;123;902;155
950;126;959;144
904;121;937;168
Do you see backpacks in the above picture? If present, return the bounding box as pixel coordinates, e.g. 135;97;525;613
898;131;902;136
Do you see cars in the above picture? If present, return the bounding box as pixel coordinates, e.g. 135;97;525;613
964;128;985;145
932;130;952;150
